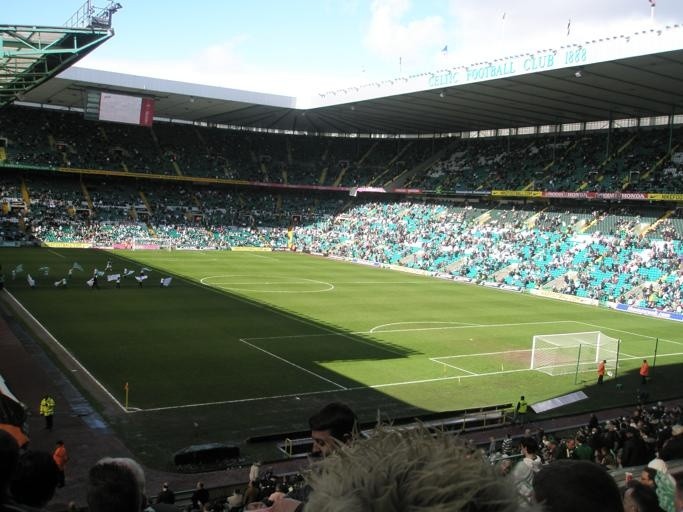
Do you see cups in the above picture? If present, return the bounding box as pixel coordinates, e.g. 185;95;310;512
626;472;633;487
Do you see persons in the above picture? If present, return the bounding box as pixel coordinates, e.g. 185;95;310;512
0;359;683;512
5;251;173;290
302;127;683;318
0;99;302;252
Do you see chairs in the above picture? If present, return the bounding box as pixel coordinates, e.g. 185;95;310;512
306;135;681;317
0;105;305;253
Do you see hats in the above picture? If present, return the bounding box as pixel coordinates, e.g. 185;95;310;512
648;459;666;475
96;457;147;510
